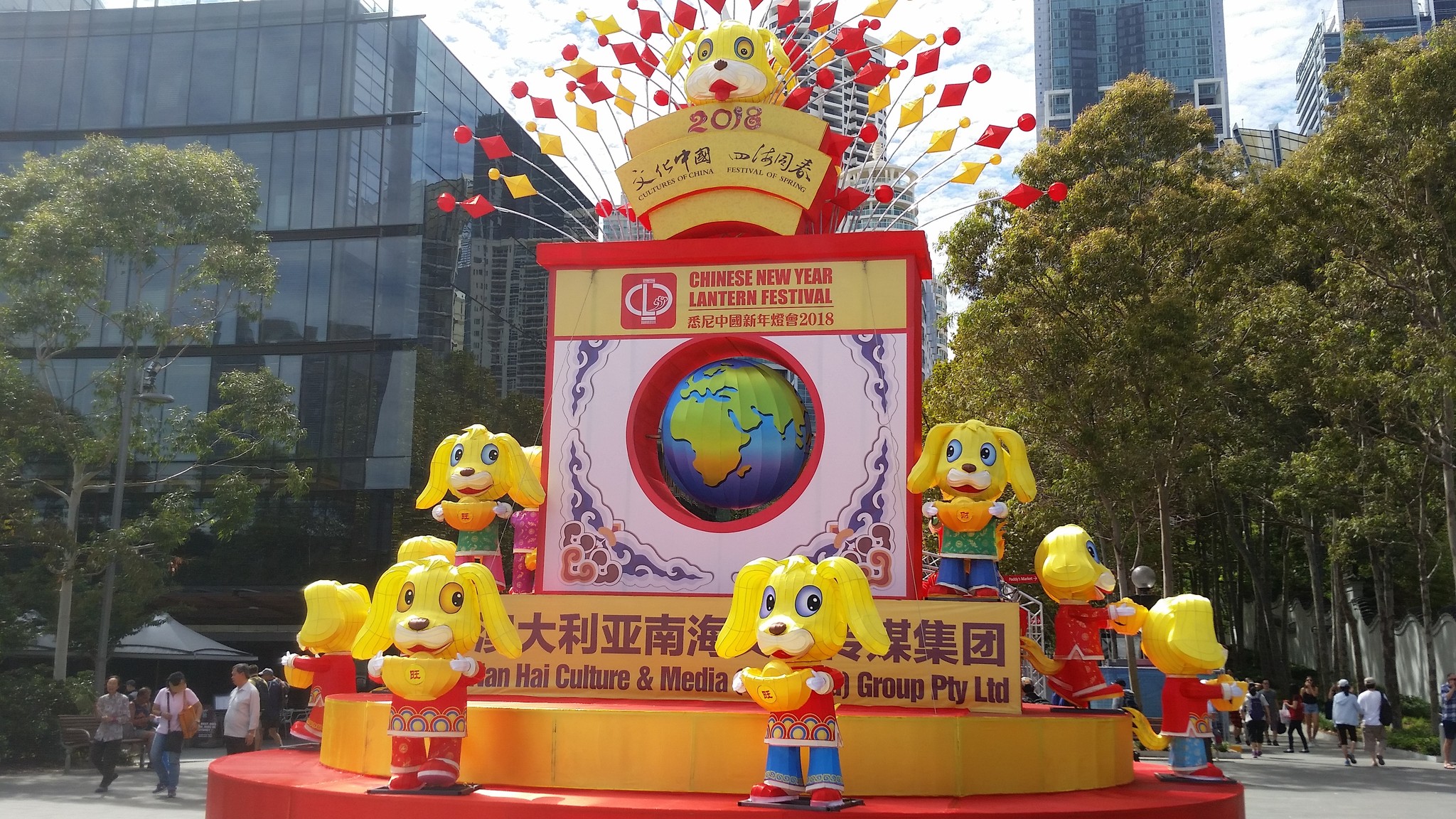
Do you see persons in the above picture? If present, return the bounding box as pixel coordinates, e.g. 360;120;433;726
1021;658;1397;778
258;668;288;747
244;665;270;742
122;687;159;762
1438;672;1456;770
149;672;204;798
89;676;131;792
223;663;260;754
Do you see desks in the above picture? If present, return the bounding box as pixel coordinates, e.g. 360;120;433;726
292;709;310;712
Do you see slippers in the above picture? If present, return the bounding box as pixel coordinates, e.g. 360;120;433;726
1444;764;1455;769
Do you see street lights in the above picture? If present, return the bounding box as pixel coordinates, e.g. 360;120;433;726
92;393;175;705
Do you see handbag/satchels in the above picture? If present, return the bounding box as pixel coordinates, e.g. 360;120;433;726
1324;700;1332;719
1279;703;1291;723
1271;720;1286;734
165;731;182;753
179;690;198;739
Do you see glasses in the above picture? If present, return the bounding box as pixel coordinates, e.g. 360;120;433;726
1306;679;1312;681
1449;678;1455;680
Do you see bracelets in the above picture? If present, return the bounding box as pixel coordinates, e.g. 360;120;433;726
249;732;256;738
196;719;201;724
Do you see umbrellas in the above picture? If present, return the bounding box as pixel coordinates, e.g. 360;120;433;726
0;607;259;711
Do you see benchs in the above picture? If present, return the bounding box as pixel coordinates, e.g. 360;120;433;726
57;714;146;771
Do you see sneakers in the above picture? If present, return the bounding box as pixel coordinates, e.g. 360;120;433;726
152;783;168;794
168;788;177;797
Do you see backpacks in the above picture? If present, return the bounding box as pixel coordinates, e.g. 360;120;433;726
1380;691;1393;726
1245;693;1265;721
280;681;289;705
250;677;269;707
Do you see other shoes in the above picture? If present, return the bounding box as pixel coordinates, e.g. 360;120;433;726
1245;740;1250;746
1284;750;1294;753
1371;762;1378;766
1345;760;1350;766
1376;752;1385;765
1267;741;1272;745
1309;738;1317;744
1252;751;1263;758
1235;737;1241;743
111;773;118;780
95;787;107;792
1347;752;1357;764
146;763;154;770
1298;749;1309;753
1273;740;1278;746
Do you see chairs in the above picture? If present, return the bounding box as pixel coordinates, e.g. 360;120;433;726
295;713;307;723
305;708;312;719
281;709;293;738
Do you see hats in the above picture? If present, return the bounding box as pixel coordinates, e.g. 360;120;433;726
1364;677;1376;685
1249;681;1256;688
1338;679;1349;687
258;668;273;675
